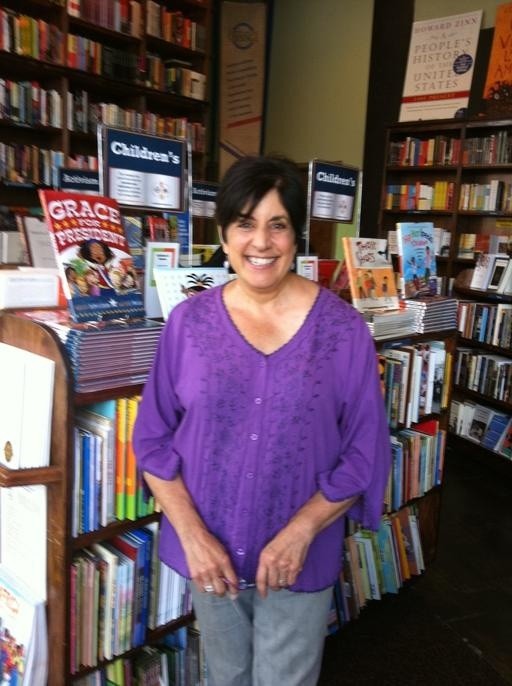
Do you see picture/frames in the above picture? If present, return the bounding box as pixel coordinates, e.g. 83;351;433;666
97;121;187;214
309;157;358;227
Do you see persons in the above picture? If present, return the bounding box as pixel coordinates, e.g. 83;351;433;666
131;157;389;684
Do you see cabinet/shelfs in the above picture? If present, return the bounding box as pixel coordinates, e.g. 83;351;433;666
0;318;458;686
380;121;512;488
1;2;221;204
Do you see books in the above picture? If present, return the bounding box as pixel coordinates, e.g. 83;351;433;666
0;484;49;610
203;585;215;594
65;624;198;686
380;132;512;213
396;221;439;298
342;236;398;309
459;227;512;459
399;9;484;126
361;296;462;339
2;590;50;686
44;294;169;396
437;227;452;257
0;202;43;261
66;519;191;668
2;71;208;155
39;0;208;53
0;139;102;189
40;190;150;322
368;347;464;508
0;341;56;469
482;1;512;123
277;578;288;589
65;397;156;540
0;4;212;103
